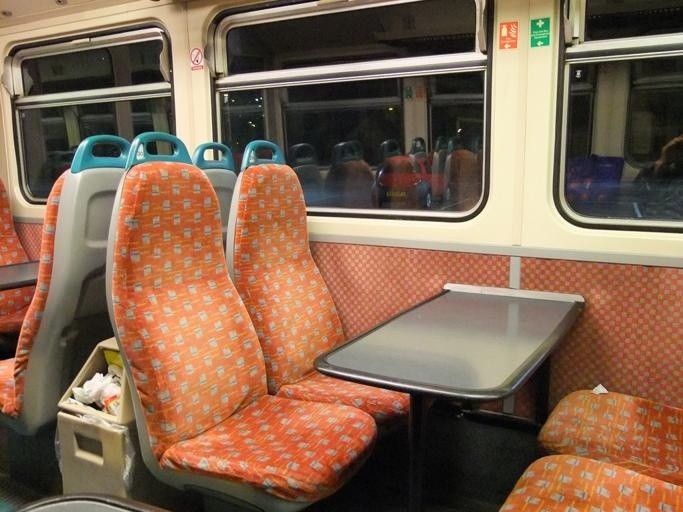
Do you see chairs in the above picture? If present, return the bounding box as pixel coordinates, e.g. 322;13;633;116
498;453;683;512
565;137;682;218
537;388;683;483
291;117;484;213
189;140;237;266
227;139;412;430
2;134;130;499
104;129;376;510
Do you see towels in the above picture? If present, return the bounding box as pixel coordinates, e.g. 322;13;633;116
55;336;143;499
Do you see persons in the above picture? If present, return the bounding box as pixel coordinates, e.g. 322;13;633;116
632;133;682;220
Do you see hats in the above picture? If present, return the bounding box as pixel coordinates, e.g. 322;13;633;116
369;154;451;210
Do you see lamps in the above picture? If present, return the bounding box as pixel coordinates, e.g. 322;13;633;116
498;453;683;512
2;134;130;499
104;129;376;510
189;140;237;266
227;139;412;430
537;388;683;483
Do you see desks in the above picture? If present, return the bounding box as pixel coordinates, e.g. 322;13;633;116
1;261;45;296
313;282;587;510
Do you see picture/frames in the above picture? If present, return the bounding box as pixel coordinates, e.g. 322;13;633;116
313;282;587;510
1;261;45;296
